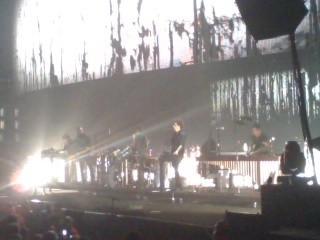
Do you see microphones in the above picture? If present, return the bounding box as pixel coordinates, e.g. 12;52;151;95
216;127;225;130
269;137;276;142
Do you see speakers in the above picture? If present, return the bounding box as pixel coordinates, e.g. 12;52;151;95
236;0;309;41
259;184;320;228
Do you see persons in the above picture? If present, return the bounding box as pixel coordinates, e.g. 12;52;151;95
1;191;236;240
60;123;152;189
166;120;188;181
250;123;276;155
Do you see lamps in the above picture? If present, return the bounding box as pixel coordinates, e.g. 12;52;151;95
279;140;307;187
